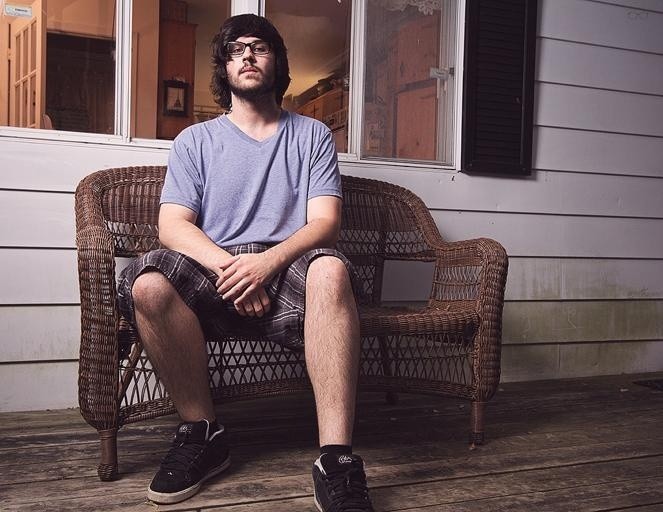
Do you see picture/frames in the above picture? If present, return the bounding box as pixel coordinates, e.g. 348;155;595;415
162;80;190;118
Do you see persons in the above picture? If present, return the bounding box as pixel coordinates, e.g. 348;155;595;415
114;13;374;511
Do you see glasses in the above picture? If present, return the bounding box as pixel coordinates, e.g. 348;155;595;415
226;40;274;55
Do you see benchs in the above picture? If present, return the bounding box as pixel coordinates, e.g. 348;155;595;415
75;165;509;482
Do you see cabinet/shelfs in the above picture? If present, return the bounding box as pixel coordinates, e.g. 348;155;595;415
158;18;199;139
390;13;438;159
291;88;348;153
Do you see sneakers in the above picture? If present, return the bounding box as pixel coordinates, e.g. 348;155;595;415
147;419;231;503
312;453;372;512
